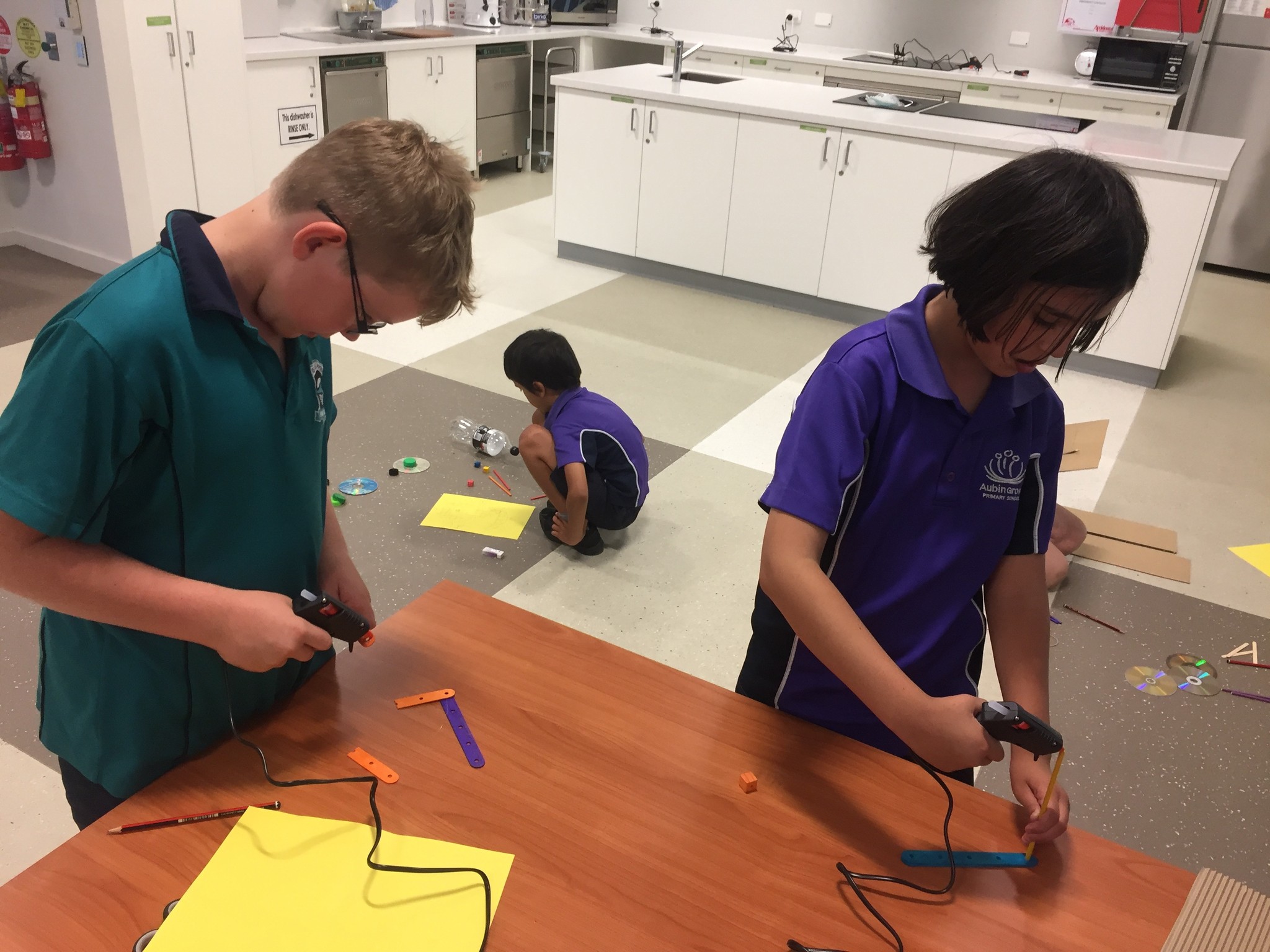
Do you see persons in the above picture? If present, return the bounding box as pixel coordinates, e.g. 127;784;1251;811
505;330;650;556
736;148;1150;844
1044;502;1088;590
0;117;481;833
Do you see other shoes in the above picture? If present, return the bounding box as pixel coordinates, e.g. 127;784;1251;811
540;507;604;555
547;499;556;508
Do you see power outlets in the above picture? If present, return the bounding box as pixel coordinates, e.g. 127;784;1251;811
647;0;662;9
785;9;802;23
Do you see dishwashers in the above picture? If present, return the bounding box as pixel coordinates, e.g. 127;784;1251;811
319;52;387;135
475;42;531;165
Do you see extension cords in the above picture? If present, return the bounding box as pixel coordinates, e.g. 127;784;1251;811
866;51;909;61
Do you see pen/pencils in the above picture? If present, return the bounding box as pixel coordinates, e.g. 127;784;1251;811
1227;660;1270;669
492;469;510;490
106;801;282;835
1221;688;1270;703
1064;604;1125;634
1049;615;1062;625
488;476;512;496
531;495;547;501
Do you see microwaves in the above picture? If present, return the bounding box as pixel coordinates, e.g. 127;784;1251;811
551;0;618;26
1090;36;1194;93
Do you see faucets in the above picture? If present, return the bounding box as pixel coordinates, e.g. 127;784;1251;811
672;39;706;82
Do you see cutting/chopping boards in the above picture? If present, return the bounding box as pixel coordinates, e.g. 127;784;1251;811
387;28;453;38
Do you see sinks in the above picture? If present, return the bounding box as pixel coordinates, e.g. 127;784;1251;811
656;70;746;85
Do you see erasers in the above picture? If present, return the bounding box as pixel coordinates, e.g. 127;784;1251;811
483;546;503;558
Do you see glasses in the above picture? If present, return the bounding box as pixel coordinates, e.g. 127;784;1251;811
318;198;378;335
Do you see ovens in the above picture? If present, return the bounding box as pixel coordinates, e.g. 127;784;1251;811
823;76;961;104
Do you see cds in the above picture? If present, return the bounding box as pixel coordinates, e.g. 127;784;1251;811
392;456;430;473
338;478;379;495
1168;666;1222;696
1124;666;1177;697
1165;653;1218;679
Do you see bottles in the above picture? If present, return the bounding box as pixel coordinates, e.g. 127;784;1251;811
450;416;520;457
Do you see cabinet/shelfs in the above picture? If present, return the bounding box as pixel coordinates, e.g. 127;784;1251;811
556;46;1227;390
120;0;478;248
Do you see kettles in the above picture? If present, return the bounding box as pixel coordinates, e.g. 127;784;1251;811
462;0;501;27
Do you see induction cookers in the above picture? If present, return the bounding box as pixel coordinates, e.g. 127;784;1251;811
843;53;961;72
919;101;1097;134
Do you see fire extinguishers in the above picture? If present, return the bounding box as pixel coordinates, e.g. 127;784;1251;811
0;59;52;171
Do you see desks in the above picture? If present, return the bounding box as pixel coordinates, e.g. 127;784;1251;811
0;578;1270;952
